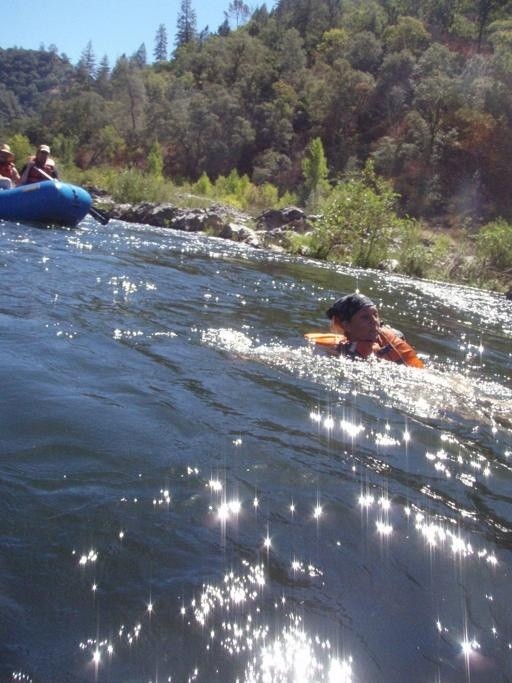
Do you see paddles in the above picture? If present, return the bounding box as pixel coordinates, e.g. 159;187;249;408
32;167;109;225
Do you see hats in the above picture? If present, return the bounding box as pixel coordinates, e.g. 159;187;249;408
0;144;14;154
39;144;50;154
326;294;374;321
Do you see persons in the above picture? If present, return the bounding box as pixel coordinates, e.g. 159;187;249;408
21;143;60;185
0;141;21;192
328;294;426;370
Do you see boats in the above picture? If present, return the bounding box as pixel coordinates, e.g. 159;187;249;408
0;179;93;227
